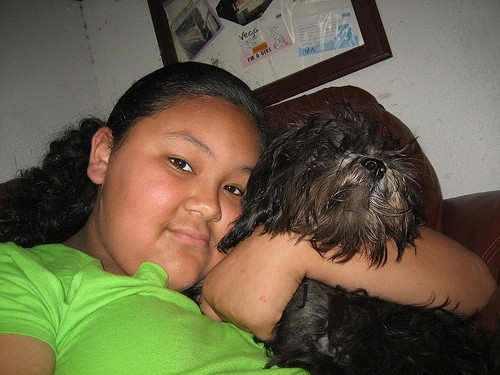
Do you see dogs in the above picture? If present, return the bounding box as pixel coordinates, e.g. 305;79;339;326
180;99;500;374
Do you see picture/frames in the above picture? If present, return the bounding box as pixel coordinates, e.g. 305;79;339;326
146;0;395;117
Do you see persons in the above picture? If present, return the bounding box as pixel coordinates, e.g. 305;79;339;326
0;61;500;375
